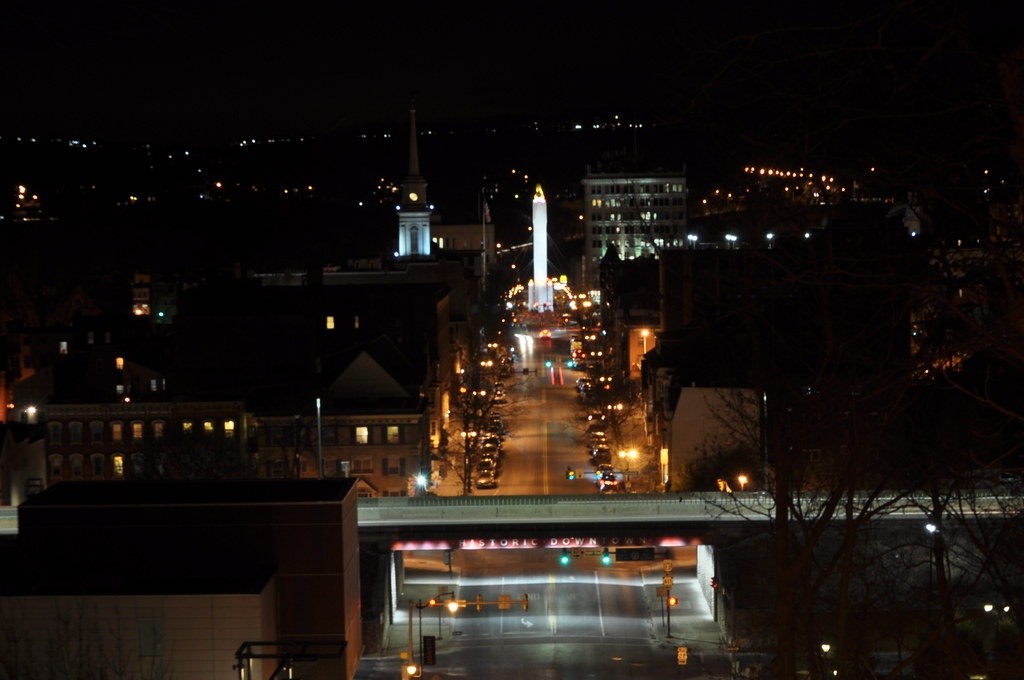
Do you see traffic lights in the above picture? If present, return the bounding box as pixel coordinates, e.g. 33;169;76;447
561;549;568;566
566;471;575;481
596;470;601;480
602;549;609;566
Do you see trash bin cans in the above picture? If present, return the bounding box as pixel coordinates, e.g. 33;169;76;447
423;636;436;665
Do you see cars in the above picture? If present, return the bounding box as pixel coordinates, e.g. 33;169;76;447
570;336;620;495
475;343;514;488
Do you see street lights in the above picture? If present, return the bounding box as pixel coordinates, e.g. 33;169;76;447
417;591;458;674
641;329;649;354
620;450;637;482
